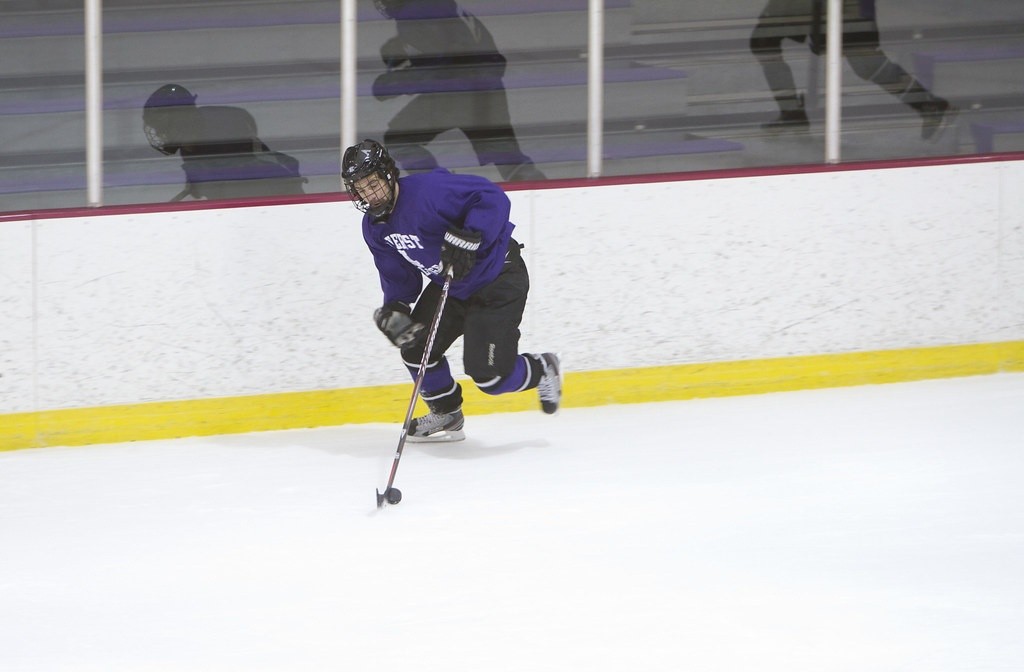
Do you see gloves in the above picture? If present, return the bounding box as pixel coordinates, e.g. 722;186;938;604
373;297;429;351
380;35;407;67
371;71;401;101
437;225;482;282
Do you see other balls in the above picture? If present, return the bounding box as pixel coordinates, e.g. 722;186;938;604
388;488;402;504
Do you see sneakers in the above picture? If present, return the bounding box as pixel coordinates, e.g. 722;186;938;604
919;94;953;142
405;397;466;443
760;91;811;134
540;352;564;414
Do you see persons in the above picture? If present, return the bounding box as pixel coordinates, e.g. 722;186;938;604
371;0;549;185
341;138;563;443
141;82;309;202
749;0;951;140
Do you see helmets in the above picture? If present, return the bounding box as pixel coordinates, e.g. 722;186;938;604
341;139;398;217
143;84;197;155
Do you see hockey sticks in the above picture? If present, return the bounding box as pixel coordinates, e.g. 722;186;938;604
375;259;455;506
